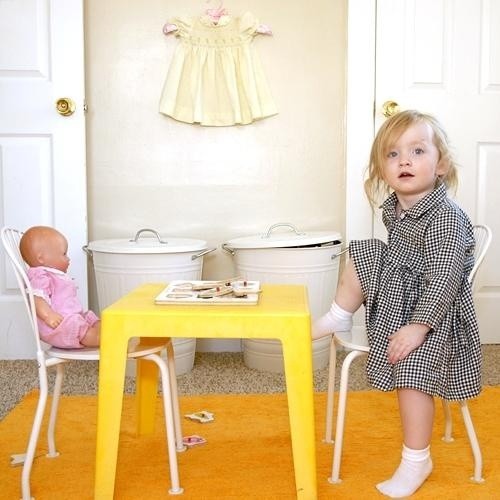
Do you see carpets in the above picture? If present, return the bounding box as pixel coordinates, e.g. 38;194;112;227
1;384;500;500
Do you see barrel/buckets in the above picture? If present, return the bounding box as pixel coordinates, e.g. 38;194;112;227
222;220;351;374
82;227;217;380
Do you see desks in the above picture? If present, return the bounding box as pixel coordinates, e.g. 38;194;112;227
94;281;318;500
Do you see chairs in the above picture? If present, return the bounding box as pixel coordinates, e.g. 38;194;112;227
322;224;493;485
1;228;187;492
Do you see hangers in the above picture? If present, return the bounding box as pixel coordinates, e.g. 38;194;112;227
163;1;271;37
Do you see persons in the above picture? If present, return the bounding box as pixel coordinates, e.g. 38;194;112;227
305;109;484;500
18;224;141;355
